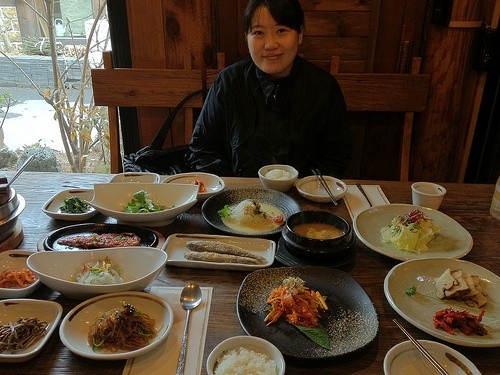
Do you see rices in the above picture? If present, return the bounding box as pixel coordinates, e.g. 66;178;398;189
263;168;295;180
213;346;278;375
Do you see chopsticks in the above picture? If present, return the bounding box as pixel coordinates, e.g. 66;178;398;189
312;168;338;206
393;319;449;375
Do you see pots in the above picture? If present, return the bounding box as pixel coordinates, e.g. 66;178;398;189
0;188;26;226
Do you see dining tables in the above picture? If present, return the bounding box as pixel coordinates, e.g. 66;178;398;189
0;171;500;375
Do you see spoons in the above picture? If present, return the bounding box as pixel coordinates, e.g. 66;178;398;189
174;282;203;375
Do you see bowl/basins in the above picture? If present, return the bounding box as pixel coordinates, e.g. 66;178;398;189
26;250;168;299
42;170;225;227
206;335;285;375
0;249;41;299
296;175;347;203
258;164;299;193
281;209;354;265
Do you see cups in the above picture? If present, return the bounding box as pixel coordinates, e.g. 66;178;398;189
411;181;447;210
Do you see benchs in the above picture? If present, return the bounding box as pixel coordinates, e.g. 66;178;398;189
91;51;430;182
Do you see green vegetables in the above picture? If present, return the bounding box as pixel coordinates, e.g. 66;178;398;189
292;323;331;351
121;190;167;214
59;197;90;213
92;309;153;352
217;204;231;218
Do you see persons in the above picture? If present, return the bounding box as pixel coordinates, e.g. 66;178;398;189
184;0;350;179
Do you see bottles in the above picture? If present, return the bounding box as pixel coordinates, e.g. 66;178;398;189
489;176;500;221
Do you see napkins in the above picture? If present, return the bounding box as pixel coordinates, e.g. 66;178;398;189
342;185;390;222
121;286;213;375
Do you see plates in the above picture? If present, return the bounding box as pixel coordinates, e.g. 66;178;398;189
275;228;356;266
383;339;482;375
58;291;174;360
161;233;276;272
201;187;301;239
383;256;500;348
353;202;474;262
0;298;63;363
236;266;380;360
42;222;159;248
